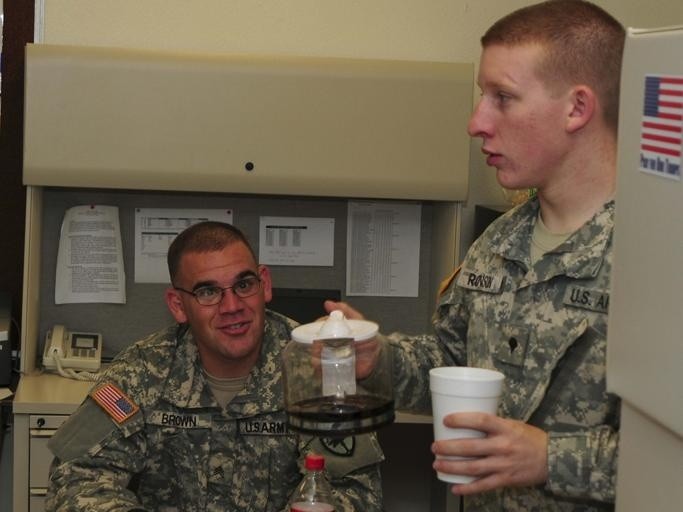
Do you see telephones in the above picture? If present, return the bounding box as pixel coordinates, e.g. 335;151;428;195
42;324;103;373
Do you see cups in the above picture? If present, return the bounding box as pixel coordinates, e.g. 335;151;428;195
429;367;506;485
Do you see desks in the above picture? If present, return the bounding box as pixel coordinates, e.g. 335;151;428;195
10;359;462;512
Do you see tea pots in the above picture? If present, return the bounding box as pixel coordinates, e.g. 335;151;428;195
280;308;395;436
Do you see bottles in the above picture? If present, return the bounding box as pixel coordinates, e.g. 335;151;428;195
291;455;336;512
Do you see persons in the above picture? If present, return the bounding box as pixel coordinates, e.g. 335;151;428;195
308;0;621;512
45;221;387;512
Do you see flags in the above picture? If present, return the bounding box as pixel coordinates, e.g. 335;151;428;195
640;73;683;181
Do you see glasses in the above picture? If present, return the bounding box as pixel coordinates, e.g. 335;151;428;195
175;272;261;306
